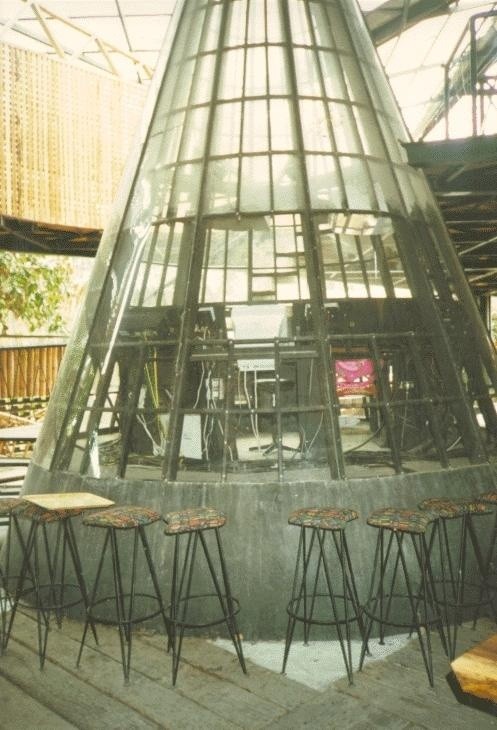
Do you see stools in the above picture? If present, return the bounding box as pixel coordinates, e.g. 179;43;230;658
164;507;247;686
3;505;98;670
78;506;169;683
419;500;493;665
459;492;497;629
248;378;299;456
280;507;368;685
360;506;449;687
0;497;49;649
